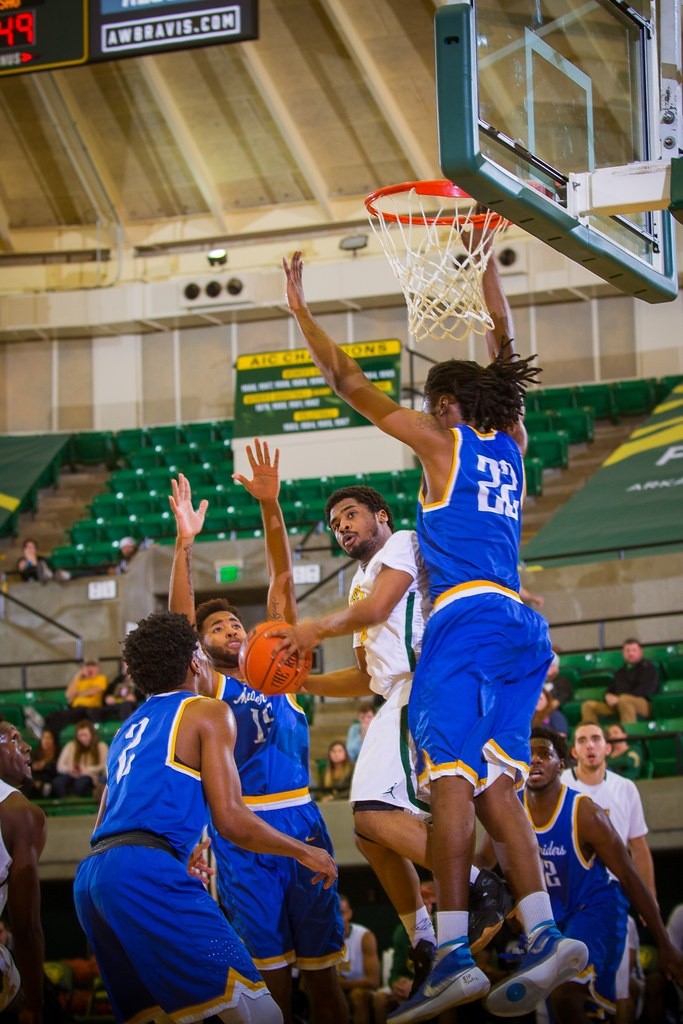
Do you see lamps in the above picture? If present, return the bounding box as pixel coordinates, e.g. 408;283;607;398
208;248;228;268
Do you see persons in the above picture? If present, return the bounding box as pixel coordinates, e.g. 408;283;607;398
52;720;109;797
168;197;683;1024
104;657;142;720
474;911;523;975
296;890;379;1018
63;653;108;722
319;740;354;802
71;607;340;1024
25;727;59;799
374;878;437;1023
114;536;154;576
0;722;46;1023
581;637;659;725
345;701;375;762
602;723;641;776
529;646;571;739
16;539;55;581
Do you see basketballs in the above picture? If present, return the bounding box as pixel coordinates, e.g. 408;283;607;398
238;620;312;695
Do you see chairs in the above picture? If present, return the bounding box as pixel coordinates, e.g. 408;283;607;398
0;372;683;817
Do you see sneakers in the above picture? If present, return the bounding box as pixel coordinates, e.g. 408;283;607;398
471;870;517;953
387;944;491;1024
487;923;589;1017
407;940;438;1000
